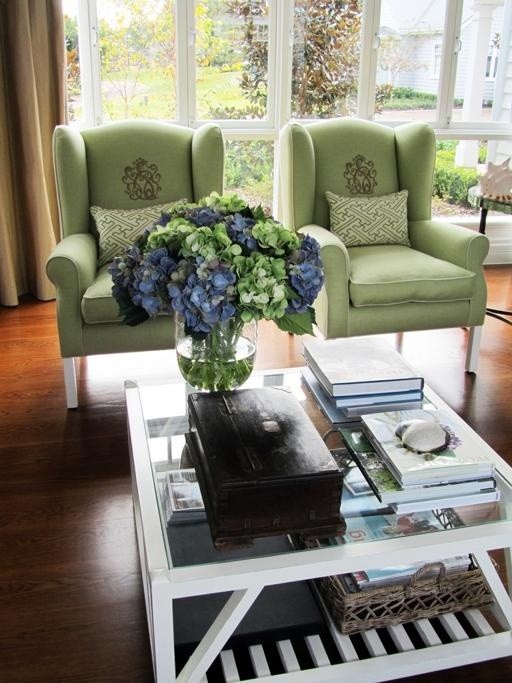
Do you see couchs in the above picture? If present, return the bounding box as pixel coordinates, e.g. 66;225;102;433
45;119;224;409
265;115;491;374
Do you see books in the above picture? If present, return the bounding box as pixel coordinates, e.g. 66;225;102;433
301;339;425;432
337;409;501;515
167;469;207;524
325;448;471;592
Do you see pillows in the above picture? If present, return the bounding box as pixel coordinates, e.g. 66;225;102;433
321;184;412;248
90;196;190;265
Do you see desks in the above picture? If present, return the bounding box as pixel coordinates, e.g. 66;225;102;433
466;182;512;326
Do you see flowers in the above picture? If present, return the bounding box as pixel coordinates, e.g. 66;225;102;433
105;186;324;387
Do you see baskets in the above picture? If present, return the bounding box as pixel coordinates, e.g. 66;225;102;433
285;429;500;635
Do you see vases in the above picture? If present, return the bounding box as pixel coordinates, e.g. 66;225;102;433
174;323;257;389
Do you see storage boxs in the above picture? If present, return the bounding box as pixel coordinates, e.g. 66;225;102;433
178;386;350;546
